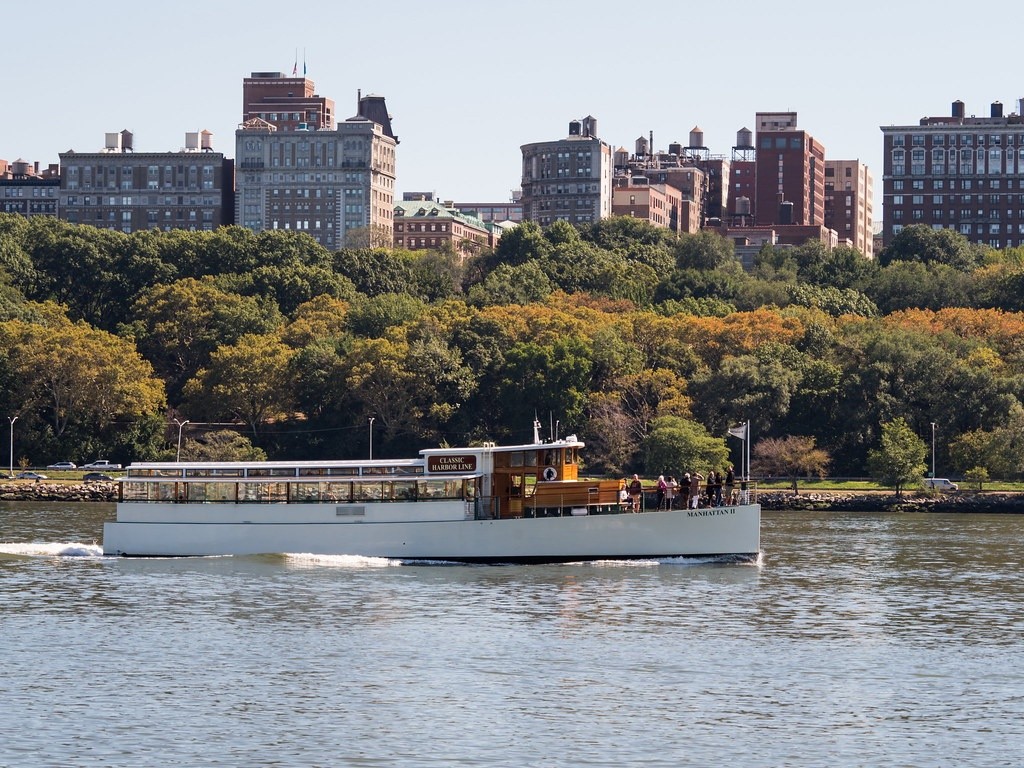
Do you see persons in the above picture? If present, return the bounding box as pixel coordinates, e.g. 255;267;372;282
544;449;556;467
622;465;734;514
421;479;478;501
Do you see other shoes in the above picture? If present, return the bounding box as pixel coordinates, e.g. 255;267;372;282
666;510;671;512
683;508;689;510
706;505;711;508
655;509;660;512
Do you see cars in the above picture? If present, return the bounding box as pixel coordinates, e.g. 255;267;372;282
0;472;11;480
82;472;114;482
16;471;47;479
47;461;76;470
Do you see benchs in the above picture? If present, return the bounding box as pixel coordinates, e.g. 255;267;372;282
586;502;631;514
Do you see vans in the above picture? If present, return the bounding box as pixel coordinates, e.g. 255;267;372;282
921;477;958;490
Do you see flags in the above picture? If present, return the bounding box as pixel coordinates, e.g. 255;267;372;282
727;424;747;439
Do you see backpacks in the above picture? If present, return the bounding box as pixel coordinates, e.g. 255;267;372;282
657;486;665;497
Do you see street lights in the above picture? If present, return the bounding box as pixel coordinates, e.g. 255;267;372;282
172;417;189;463
929;421;936;477
7;415;17;476
368;417;374;459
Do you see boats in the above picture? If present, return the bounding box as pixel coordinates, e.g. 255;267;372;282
98;432;762;561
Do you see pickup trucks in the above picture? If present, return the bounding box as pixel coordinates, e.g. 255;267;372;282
85;460;122;471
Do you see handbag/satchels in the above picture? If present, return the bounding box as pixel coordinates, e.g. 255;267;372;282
672;487;678;494
